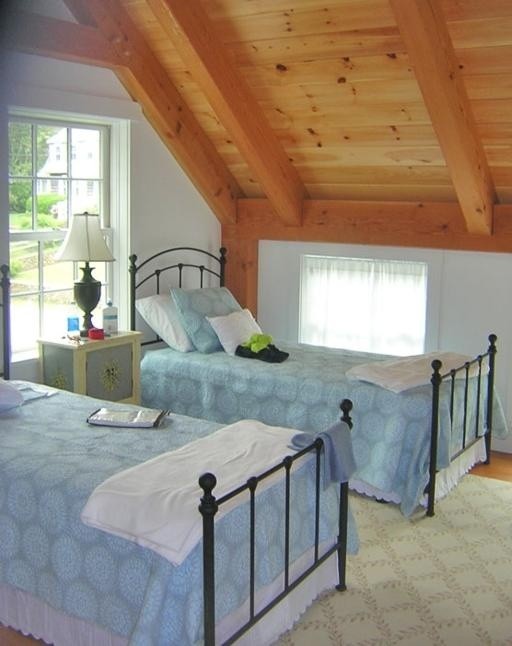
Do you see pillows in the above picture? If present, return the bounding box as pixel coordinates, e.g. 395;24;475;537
134;292;196;352
170;287;242;352
204;308;264;354
0;376;25;410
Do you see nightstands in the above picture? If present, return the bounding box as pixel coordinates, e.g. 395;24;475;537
36;330;141;407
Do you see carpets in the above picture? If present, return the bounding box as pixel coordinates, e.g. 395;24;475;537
274;472;512;646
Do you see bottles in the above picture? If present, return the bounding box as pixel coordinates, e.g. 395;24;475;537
103;299;119;335
66;300;81;344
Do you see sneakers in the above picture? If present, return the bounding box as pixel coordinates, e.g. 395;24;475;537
236;334;289;362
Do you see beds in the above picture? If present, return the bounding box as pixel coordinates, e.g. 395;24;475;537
0;264;354;645
129;245;497;517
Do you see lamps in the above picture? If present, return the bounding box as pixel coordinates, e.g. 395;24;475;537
55;210;117;335
